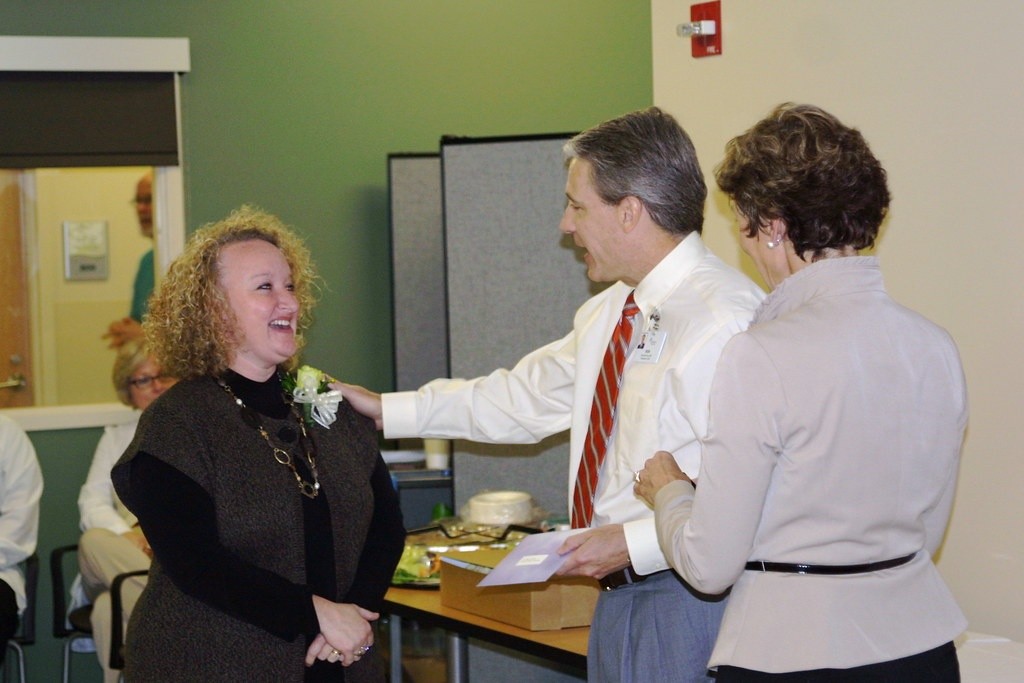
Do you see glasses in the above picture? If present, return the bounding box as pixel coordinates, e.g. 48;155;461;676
128;368;174;390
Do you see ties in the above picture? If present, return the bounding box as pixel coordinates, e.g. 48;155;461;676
570;288;641;529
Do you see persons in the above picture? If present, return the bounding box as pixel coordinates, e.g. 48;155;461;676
316;109;769;683
632;101;969;683
0;411;46;669
76;333;181;682
111;202;410;683
99;170;155;355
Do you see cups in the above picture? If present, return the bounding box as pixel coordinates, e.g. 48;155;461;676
423;439;450;471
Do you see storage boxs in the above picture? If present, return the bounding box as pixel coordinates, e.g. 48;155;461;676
437;546;603;632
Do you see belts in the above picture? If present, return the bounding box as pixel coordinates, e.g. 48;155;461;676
598;565;647;591
744;551;918;575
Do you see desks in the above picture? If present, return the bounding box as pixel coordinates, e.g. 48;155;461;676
373;518;593;683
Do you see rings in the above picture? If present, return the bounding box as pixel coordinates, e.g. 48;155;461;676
358;650;362;656
360;646;370;651
633;470;641;484
332;649;343;658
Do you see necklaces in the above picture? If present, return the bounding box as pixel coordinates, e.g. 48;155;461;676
212;369;321;500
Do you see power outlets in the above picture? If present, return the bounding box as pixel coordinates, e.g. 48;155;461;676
676;0;722;58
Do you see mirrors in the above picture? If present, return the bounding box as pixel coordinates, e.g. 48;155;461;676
1;36;193;438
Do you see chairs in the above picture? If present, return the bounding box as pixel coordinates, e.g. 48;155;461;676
108;568;153;683
5;551;38;683
49;544;109;683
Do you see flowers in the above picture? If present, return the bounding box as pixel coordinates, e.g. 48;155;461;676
279;362;342;428
